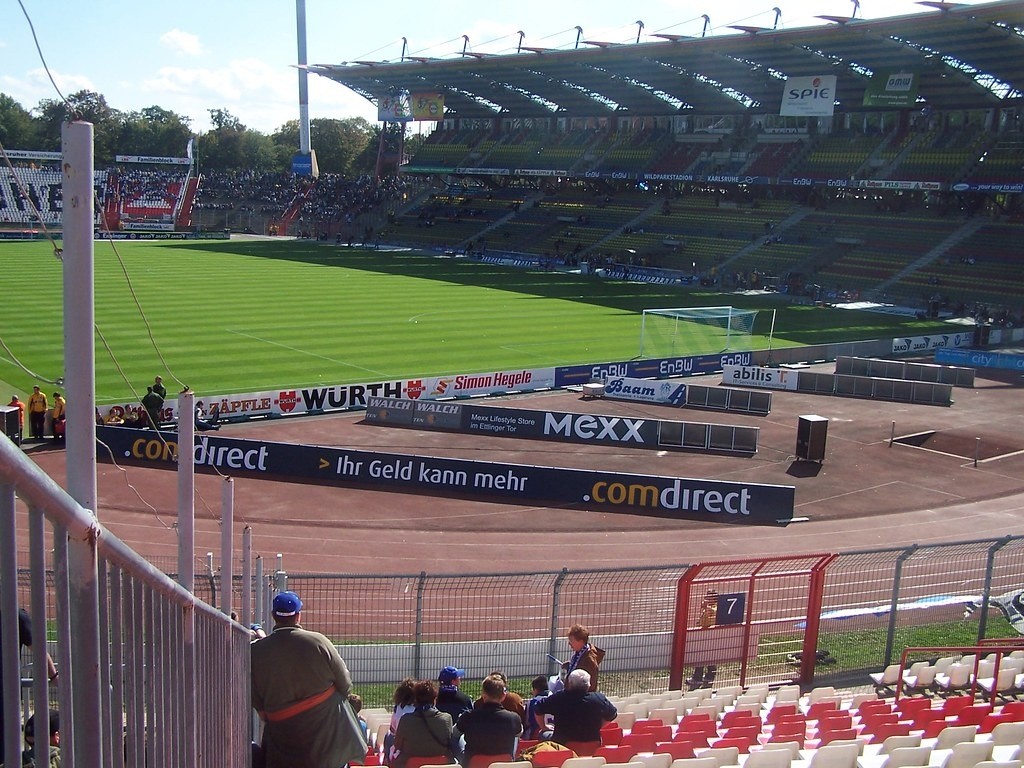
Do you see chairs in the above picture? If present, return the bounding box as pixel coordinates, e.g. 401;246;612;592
0;126;1024;311
348;654;1024;768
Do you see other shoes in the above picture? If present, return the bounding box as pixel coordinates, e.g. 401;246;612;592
702;674;714;682
686;675;702;684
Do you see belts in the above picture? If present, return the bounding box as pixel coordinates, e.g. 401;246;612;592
258;684;337;722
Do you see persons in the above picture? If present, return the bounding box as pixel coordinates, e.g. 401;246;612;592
0;609;59;768
250;590;369;768
22;709;60;768
8;161;1023;302
7;385;65;444
914;292;1024;329
686;589;719;685
95;376;167;431
381;622;618;768
231;611;266;638
194;401;221;431
347;694;367;744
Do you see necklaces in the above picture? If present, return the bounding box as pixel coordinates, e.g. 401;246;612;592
49;670;58;682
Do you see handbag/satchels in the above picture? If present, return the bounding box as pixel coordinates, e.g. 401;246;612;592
449;737;462;756
548;665;567;694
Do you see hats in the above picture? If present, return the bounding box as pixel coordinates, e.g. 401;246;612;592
24;709;60;746
438;666;464;683
273;591;303;617
